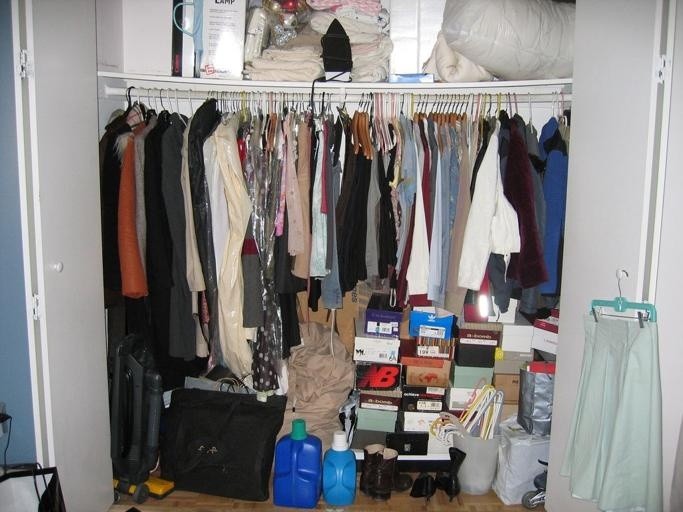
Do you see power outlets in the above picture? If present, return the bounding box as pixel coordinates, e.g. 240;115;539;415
0;401;9;437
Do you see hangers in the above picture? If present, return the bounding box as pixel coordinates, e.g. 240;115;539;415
106;86;567;160
590;269;657;328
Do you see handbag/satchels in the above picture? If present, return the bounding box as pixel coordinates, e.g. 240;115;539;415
158;376;288;502
519;360;555;436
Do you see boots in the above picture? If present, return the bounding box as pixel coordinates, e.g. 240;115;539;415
359;443;414;502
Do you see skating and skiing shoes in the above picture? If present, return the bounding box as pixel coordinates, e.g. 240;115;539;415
522;470;546;509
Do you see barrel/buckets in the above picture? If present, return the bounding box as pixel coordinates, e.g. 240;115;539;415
322;430;356;505
453;433;497;495
273;419;323;509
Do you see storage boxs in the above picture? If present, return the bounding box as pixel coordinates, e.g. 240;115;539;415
348;291;558;457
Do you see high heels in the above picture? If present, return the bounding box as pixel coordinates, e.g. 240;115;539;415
409;474;460;502
